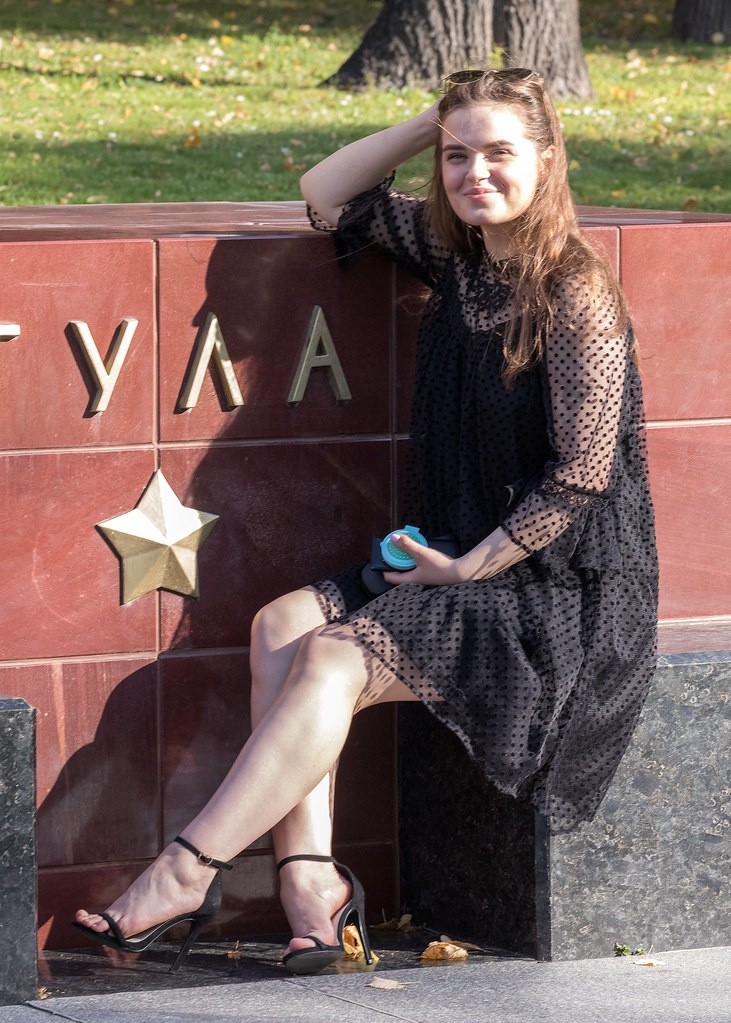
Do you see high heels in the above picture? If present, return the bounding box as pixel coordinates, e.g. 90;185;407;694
71;838;233;973
275;856;372;973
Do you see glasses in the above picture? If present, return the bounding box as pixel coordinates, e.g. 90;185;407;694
444;67;543;95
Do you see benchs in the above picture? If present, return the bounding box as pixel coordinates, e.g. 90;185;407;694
0;695;41;1007
394;621;731;964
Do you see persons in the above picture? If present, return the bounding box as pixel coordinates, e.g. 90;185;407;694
70;68;659;975
